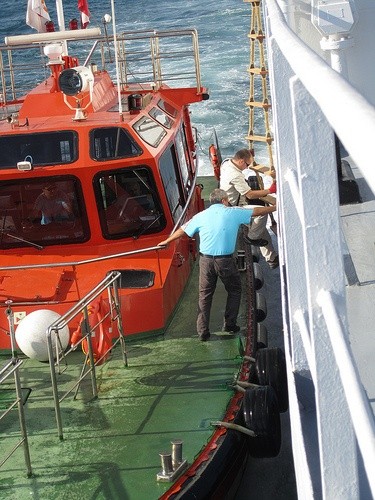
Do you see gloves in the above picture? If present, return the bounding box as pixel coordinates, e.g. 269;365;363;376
269;183;276;193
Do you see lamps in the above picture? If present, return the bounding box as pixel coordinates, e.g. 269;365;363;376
18;156;33;171
57;65;95;120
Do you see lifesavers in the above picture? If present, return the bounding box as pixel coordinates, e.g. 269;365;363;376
83;301;112;366
241;385;281;460
210;144;221;180
247;176;264;205
256;346;289;413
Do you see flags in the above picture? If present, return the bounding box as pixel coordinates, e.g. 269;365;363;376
26;0;52;33
78;0;91;29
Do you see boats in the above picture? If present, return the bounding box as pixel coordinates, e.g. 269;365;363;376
0;0;270;500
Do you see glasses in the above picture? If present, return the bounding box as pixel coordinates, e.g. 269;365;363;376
245;161;250;167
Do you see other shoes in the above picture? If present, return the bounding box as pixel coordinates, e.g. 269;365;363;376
223;326;240;332
200;332;210;341
243;236;269;246
268;256;279;269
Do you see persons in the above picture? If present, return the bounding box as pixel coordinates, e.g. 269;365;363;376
219;149;278;270
157;188;276;340
22;181;72;228
